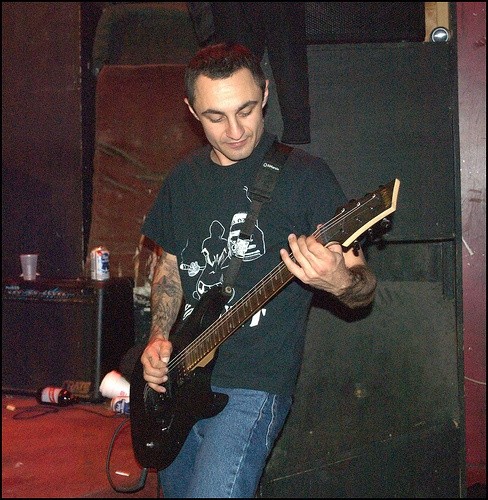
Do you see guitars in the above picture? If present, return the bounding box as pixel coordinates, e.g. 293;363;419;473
130;176;403;472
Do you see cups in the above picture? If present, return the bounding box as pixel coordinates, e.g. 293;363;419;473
99;368;131;401
110;395;130;415
20;253;39;280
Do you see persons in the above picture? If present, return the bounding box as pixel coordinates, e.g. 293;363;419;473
139;43;376;497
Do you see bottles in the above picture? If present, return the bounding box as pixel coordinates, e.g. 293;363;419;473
35;387;78;405
91;243;110;281
